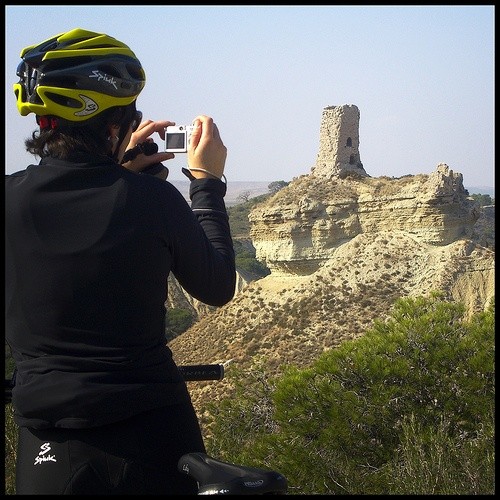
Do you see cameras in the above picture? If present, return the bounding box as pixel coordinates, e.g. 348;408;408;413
164;125;194;152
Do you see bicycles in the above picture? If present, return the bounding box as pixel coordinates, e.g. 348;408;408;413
5;364;288;495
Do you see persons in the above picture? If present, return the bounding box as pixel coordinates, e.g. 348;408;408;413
5;28;237;494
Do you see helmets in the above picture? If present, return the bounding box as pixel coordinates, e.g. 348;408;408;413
12;28;147;122
